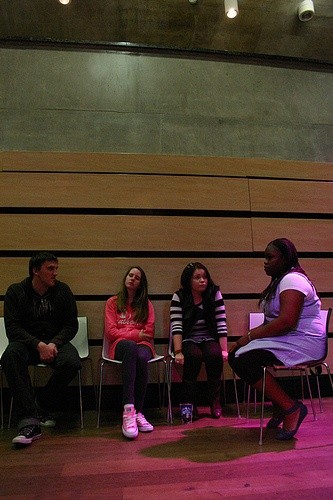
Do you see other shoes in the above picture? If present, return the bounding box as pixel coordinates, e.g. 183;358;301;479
213;407;223;417
266;402;284;429
276;400;308;441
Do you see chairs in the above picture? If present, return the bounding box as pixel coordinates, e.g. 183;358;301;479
166;322;242;425
0;316;10;432
244;311;309;423
7;316;97;434
97;322;168;430
258;308;333;446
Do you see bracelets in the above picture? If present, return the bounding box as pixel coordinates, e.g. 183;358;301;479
248;332;251;342
174;350;182;356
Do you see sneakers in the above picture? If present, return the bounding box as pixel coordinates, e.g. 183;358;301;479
121;403;137;438
136;415;154;432
35;413;56;427
10;425;40;444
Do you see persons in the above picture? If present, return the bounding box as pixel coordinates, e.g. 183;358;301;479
0;252;81;447
228;238;326;441
104;267;155;439
169;262;229;418
